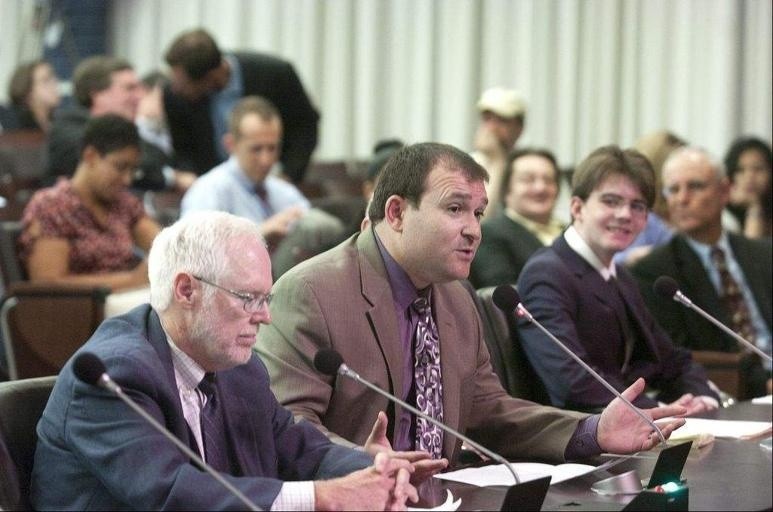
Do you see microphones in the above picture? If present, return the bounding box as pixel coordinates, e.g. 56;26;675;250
70;352;117;392
651;274;694;308
314;347;350;377
486;281;534;324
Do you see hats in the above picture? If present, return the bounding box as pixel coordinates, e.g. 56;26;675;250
476;88;526;120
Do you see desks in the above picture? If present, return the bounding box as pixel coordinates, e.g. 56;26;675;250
401;397;773;512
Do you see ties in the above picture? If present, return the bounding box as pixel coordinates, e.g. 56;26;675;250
607;275;620;305
198;371;233;475
413;293;445;458
709;246;755;357
255;185;268;203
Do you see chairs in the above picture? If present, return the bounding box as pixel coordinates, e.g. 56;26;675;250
476;284;536;401
0;376;58;512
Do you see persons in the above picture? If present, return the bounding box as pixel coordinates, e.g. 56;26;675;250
512;144;723;418
251;141;688;484
25;210;421;511
360;86;771;288
8;28;319;295
635;143;772;399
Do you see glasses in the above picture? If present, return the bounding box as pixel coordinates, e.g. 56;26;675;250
192;276;276;314
